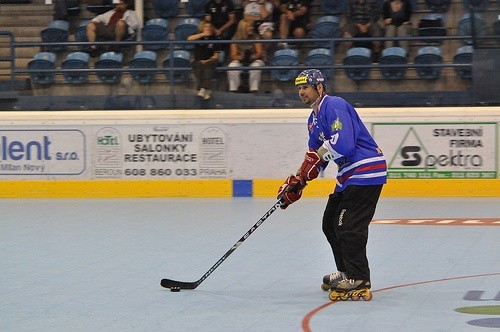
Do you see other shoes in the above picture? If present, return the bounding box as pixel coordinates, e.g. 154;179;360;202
196;90;211;100
81;46;98;54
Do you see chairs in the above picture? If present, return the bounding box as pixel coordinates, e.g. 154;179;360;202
25;0;500;88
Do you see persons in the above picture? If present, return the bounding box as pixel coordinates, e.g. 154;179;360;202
53;0;412;102
276;70;387;292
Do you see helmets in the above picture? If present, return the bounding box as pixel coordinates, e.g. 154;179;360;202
294;69;328;87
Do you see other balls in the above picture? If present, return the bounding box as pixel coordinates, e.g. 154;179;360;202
171;286;180;292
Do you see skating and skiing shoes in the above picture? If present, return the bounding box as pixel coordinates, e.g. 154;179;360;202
321;270;348;291
328;278;373;301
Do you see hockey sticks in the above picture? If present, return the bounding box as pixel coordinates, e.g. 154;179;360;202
161;179;306;290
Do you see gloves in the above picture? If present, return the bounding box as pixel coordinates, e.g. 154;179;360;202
300;151;324;186
276;176;307;209
